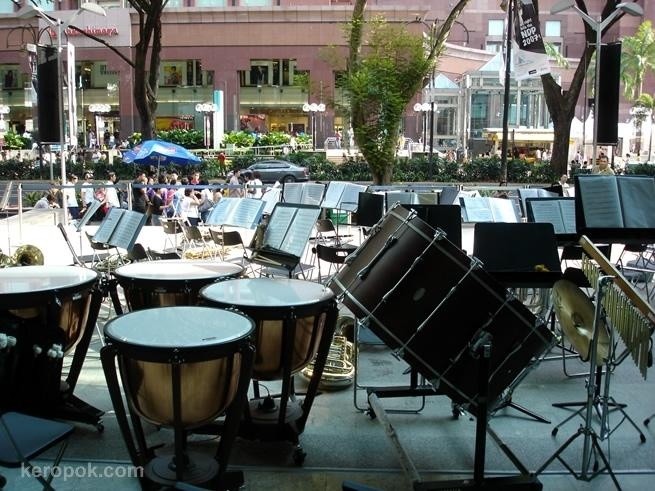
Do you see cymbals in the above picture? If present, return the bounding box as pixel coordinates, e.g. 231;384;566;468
551;280;613;365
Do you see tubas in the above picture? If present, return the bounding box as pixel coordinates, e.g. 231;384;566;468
300;303;364;387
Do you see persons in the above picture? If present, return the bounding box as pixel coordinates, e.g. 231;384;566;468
347;127;356;149
422;145;641;172
30;126;142;167
31;166;264;233
216;150;227;177
272;124;312;139
166;64;182;85
333;130;343;150
591;155;616;175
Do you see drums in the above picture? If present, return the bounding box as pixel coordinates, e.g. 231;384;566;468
200;278;335;466
103;307;257;491
115;260;245;311
1;265;106;433
326;202;559;415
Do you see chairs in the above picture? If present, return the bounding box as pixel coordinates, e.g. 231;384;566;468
85;218;358;284
542;267;617;377
0;412;75;491
353;314;425;413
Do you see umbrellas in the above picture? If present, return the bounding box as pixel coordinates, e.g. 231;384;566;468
119;138;203;179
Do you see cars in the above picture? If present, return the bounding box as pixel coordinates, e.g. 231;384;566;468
226;160;311;183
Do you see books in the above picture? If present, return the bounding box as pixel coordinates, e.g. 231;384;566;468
76;176;654;258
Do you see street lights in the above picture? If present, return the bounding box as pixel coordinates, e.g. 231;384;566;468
550;0;644;169
88;104;111;146
413;103;437;151
195;103;218;149
13;2;106;224
303;103;325;151
